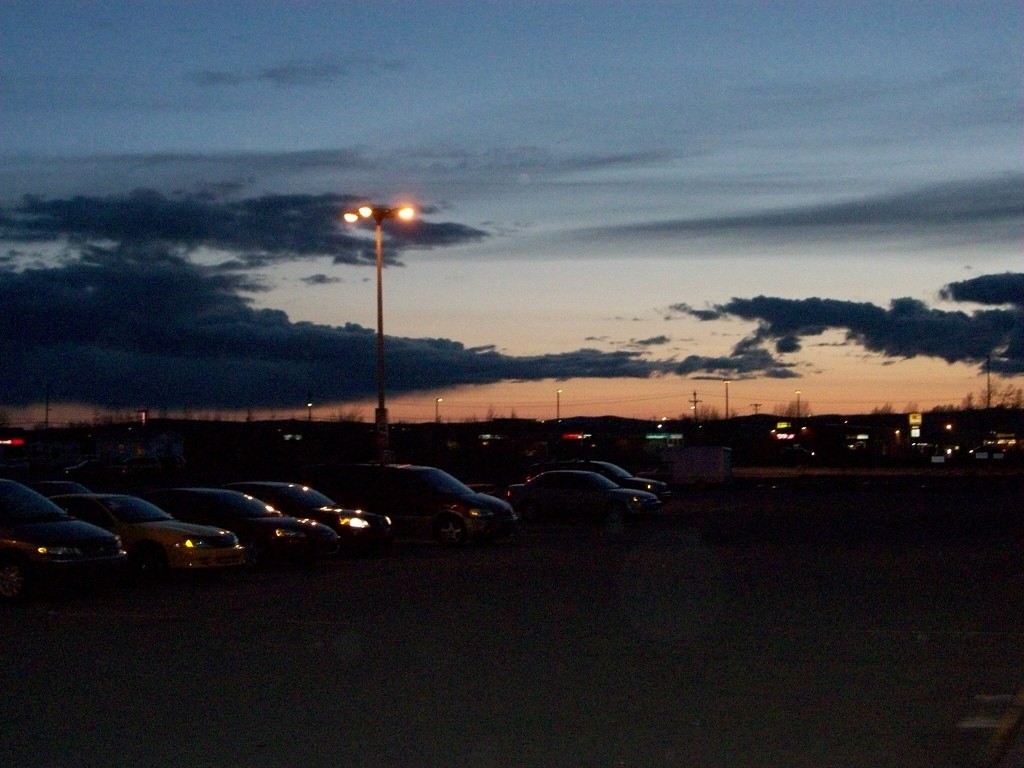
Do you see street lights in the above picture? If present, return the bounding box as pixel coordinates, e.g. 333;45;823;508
556;389;563;419
344;199;421;454
308;403;312;421
795;390;802;417
723;379;731;420
435;398;443;423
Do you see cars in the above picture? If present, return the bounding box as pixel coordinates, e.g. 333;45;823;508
29;480;93;498
0;477;130;609
308;464;521;549
226;480;392;551
64;456;124;482
46;493;248;597
505;470;663;535
533;460;672;508
155;486;342;586
